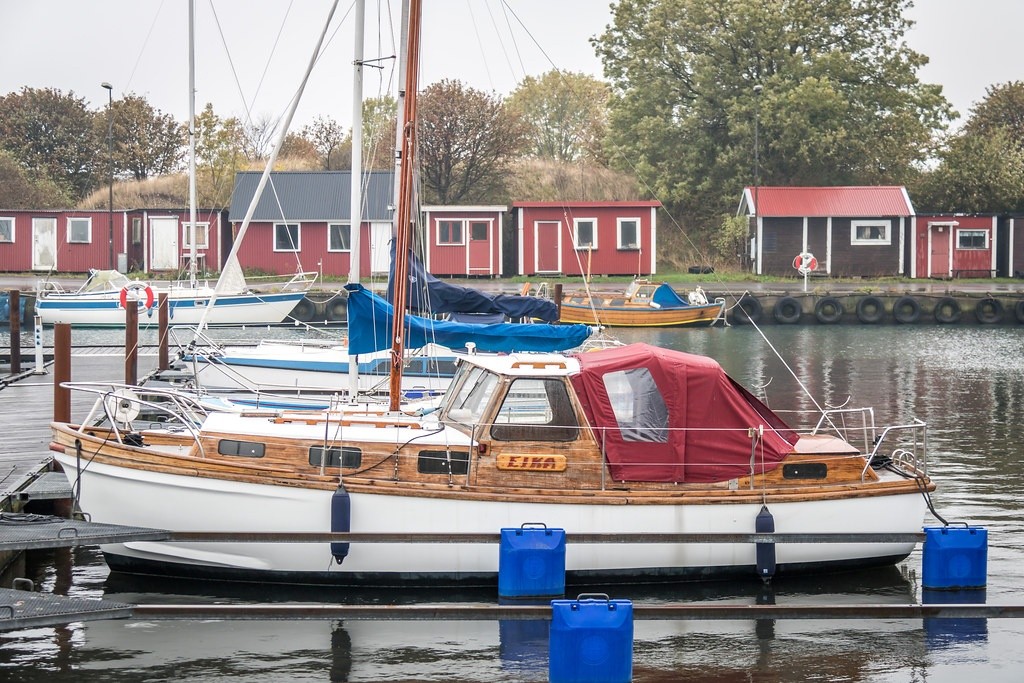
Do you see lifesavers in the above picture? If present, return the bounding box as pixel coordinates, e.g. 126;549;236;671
120;281;155;315
795;253;817;274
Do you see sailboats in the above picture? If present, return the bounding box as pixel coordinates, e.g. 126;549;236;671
31;0;944;588
